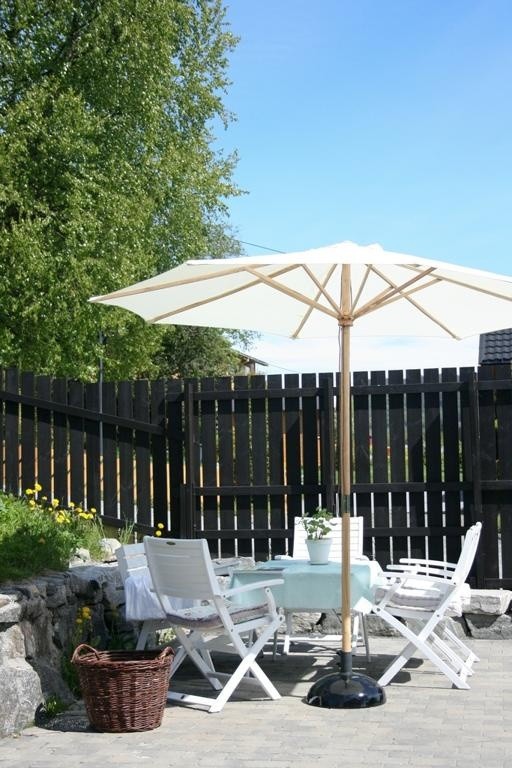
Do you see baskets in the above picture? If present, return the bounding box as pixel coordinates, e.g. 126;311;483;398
69;643;177;733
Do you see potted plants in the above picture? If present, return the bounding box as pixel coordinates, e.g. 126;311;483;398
296;504;337;564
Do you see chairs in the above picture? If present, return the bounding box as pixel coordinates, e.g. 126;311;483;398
116;543;217;675
283;516;371;663
372;526;472;690
377;521;484;675
142;535;285;713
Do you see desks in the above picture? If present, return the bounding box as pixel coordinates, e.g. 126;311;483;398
230;558;378;657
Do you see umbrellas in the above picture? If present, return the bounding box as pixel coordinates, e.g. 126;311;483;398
85;239;511;671
